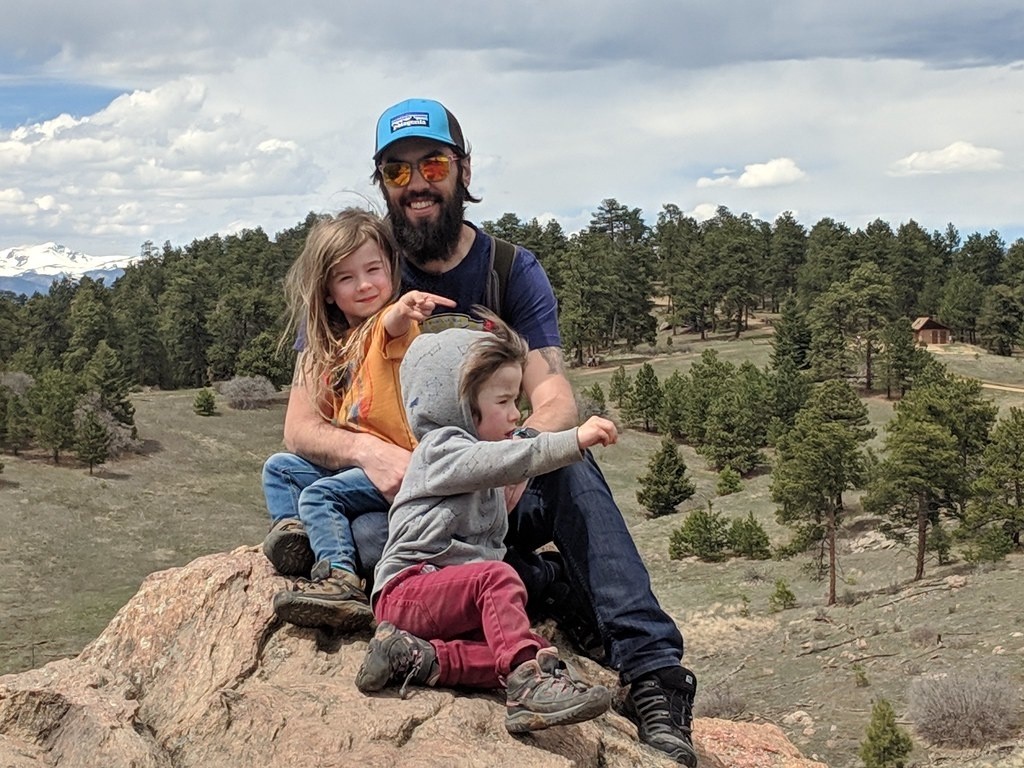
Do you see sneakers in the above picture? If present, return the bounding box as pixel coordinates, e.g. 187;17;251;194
354;620;435;693
621;666;698;767
273;566;371;628
505;646;612;735
264;518;313;575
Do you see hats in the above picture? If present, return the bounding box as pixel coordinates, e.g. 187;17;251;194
370;98;465;161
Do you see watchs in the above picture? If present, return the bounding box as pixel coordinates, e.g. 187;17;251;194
514;424;541;439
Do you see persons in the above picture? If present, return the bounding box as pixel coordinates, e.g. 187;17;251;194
261;210;459;630
355;327;617;734
280;99;700;768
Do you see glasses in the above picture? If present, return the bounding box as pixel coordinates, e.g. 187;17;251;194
376;154;458;189
